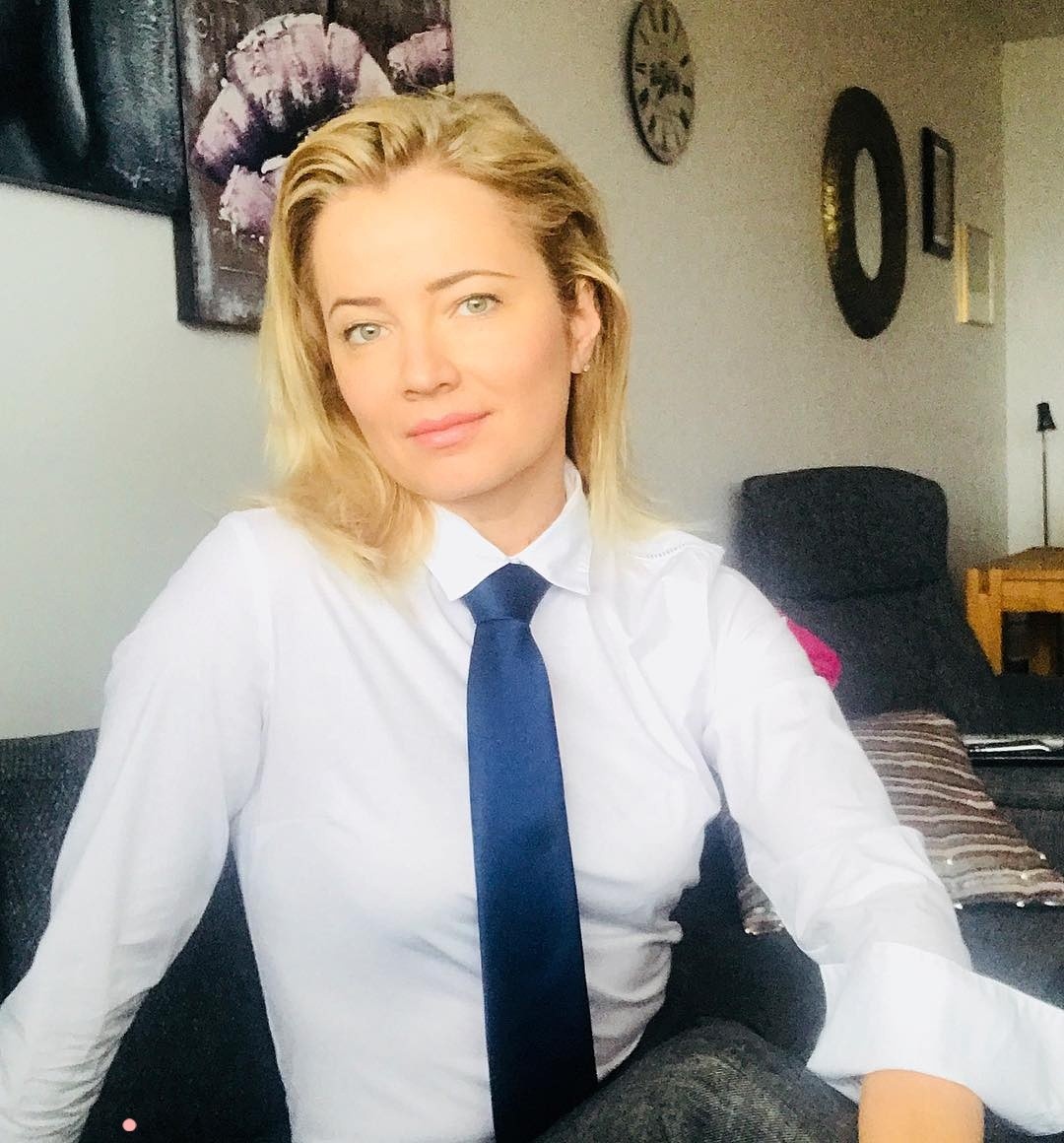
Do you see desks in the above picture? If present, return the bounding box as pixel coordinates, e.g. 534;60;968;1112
963;545;1064;676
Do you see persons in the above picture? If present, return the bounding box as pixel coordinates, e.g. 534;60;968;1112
0;92;1064;1143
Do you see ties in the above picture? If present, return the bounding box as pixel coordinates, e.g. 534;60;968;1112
464;560;599;1142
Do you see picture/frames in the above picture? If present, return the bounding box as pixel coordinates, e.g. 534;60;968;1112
957;220;997;332
917;124;957;262
810;76;911;342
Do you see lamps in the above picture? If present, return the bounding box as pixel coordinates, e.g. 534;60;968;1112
1035;400;1058;549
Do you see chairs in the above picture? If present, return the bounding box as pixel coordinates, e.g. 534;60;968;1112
731;462;1064;738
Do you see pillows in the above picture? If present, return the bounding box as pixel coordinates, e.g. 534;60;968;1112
713;702;1064;938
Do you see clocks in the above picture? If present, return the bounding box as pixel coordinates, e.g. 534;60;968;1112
626;0;697;169
